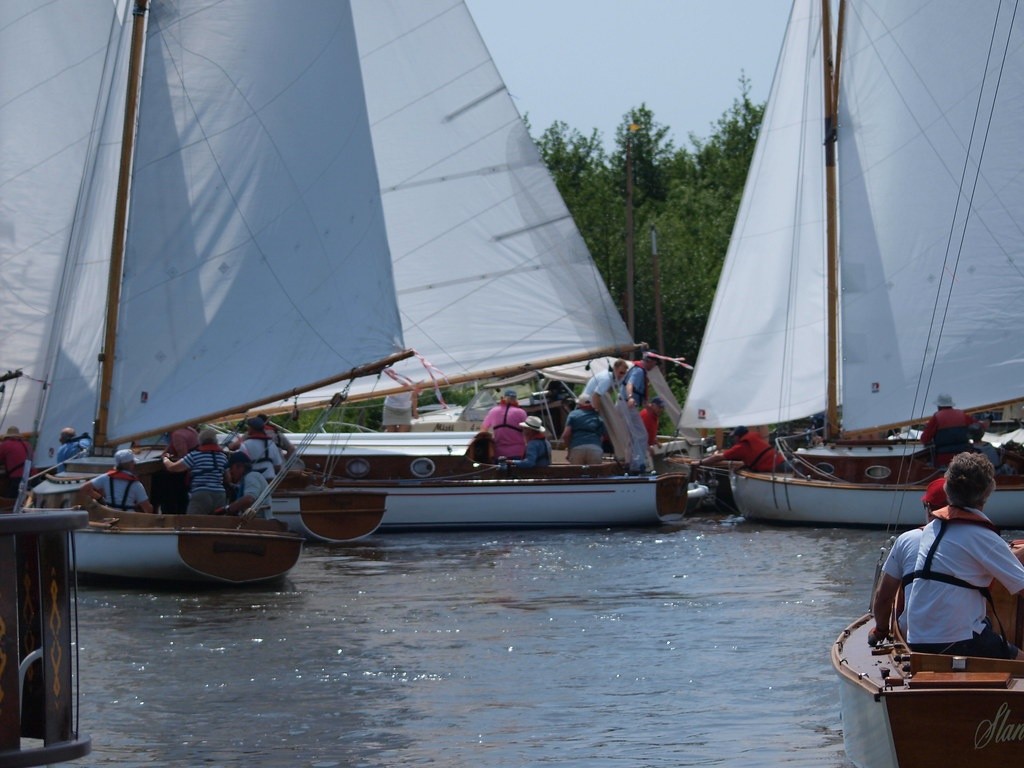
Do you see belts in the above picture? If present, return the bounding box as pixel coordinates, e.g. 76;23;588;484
619;397;629;401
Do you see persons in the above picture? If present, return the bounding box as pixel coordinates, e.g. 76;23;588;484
867;452;1024;661
617;354;662;475
468;389;552;471
56;427;93;475
167;414;295;521
575;359;629;413
690;426;794;474
640;397;665;471
561;392;608;465
920;393;987;469
968;423;1002;475
80;449;153;514
0;425;37;509
161;429;228;516
383;389;419;433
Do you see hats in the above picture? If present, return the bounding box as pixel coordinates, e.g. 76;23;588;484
920;478;949;507
247;417;264;431
223;451;251;469
651;397;666;409
115;449;140;465
574;393;593;405
519;415;546;432
4;426;24;438
504;389;517;398
728;426;748;437
197;428;221;444
934;393;956;407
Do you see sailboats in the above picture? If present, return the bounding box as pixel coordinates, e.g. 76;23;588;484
676;1;1024;531
0;0;689;595
826;3;1022;768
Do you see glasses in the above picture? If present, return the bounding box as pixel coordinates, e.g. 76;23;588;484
619;371;626;375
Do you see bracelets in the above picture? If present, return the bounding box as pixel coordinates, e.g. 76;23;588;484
700;460;703;465
626;394;633;400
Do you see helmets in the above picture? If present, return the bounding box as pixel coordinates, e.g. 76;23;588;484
966;422;985;440
